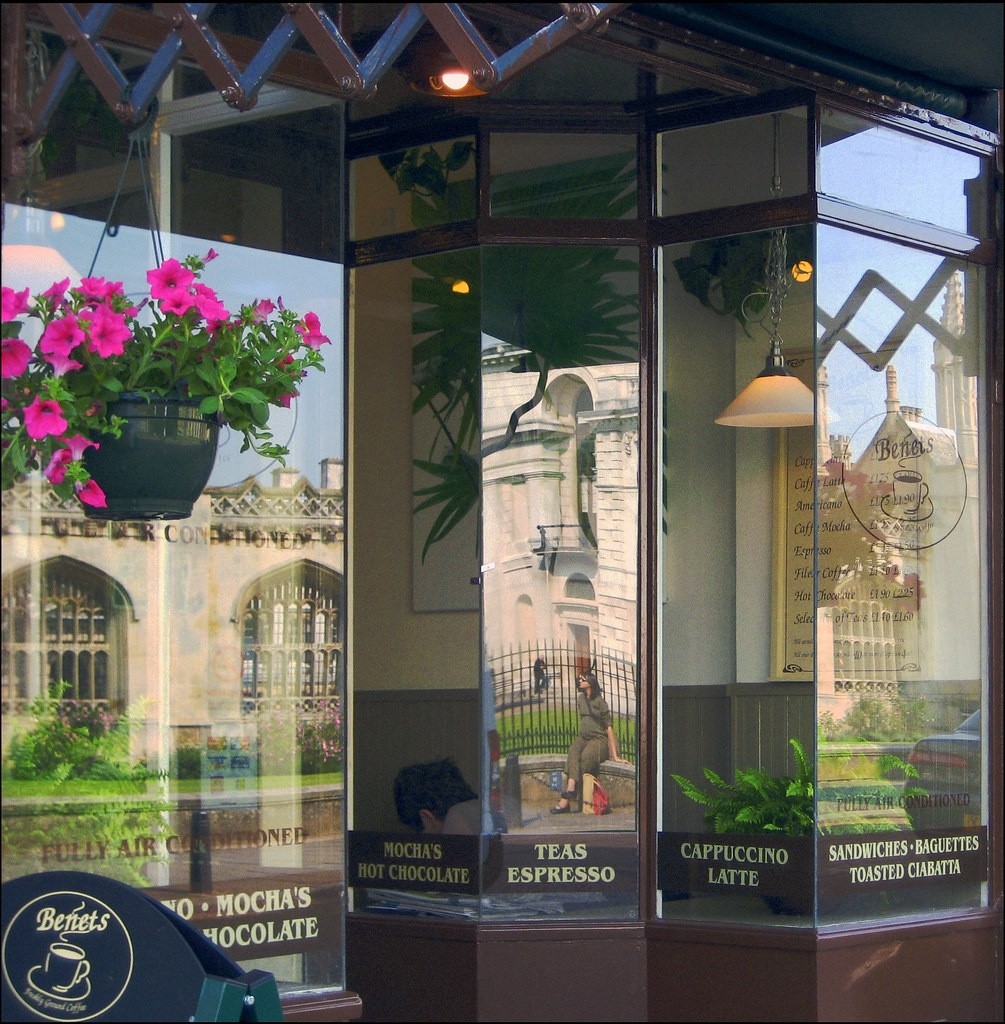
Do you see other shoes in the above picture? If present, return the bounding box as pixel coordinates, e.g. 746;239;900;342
561;789;577;799
550;803;570;814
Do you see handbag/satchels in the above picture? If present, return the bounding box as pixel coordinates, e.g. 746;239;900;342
592;779;610;815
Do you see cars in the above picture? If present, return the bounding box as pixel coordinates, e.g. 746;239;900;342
899;705;983;878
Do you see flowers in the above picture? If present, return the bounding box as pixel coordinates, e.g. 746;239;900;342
0;246;335;509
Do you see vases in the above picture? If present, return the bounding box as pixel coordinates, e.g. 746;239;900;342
73;390;220;520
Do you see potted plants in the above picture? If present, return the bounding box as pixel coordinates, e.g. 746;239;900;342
669;726;929;917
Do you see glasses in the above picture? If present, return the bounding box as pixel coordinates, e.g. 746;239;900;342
578;677;587;682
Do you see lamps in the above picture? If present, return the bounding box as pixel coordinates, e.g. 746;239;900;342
712;113;841;428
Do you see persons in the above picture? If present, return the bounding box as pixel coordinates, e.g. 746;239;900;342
534;653;548;697
550;673;631;814
393;757;511;894
